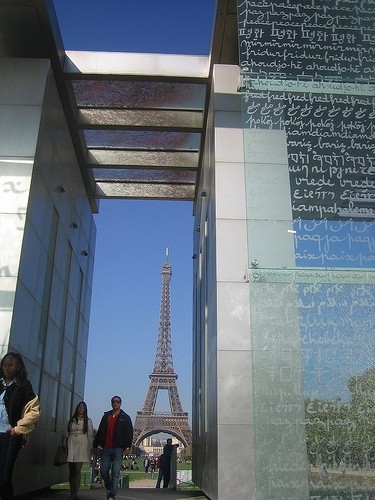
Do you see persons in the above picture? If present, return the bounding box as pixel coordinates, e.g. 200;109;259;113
93;396;133;500
53;401;94;500
143;438;179;488
122;460;139;471
0;352;41;500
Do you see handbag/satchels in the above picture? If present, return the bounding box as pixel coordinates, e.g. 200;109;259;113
53;430;69;465
156;454;163;468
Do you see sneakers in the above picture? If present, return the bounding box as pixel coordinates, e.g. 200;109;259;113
106;487;115;500
